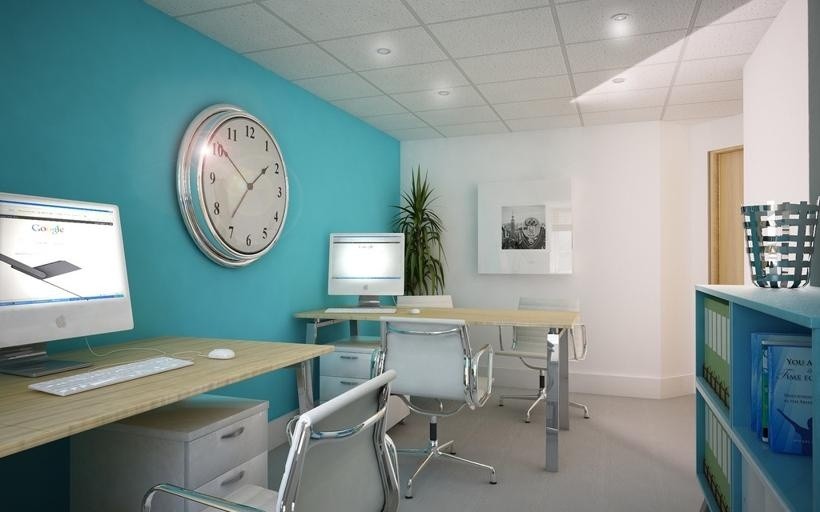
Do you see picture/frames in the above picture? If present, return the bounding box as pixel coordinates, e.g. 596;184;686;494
476;177;573;276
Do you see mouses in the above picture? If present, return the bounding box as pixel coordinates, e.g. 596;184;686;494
208;349;236;360
408;309;420;314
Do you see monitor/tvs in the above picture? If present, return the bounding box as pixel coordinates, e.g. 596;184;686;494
0;192;135;378
328;233;406;308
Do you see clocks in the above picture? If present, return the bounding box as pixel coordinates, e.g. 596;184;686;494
174;101;292;271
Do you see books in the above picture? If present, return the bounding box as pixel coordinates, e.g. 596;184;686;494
700;296;812;511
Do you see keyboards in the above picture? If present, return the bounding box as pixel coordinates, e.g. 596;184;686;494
27;356;195;397
324;308;398;314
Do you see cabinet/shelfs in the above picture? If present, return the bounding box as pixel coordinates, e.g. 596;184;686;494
318;335;410;433
695;283;819;512
69;394;270;512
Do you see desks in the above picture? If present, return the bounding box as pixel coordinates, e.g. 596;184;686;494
293;308;580;472
0;334;335;462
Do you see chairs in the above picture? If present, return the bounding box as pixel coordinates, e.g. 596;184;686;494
495;295;592;424
140;370;402;511
369;316;497;498
